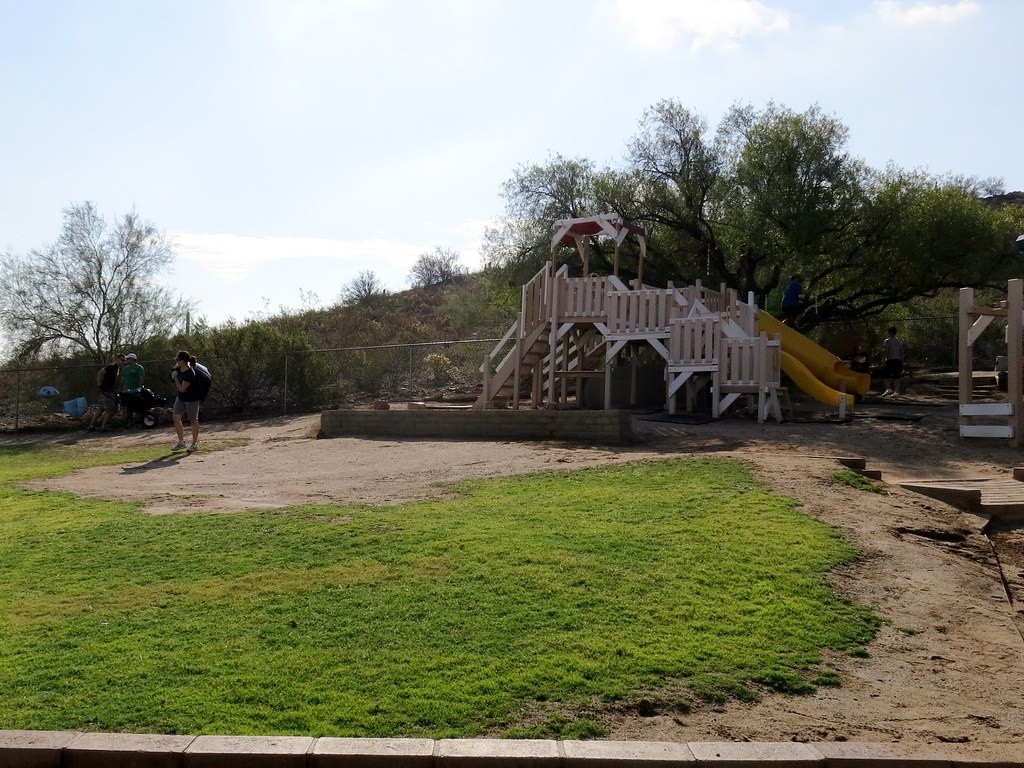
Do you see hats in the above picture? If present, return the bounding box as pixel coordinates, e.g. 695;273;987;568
125;353;137;360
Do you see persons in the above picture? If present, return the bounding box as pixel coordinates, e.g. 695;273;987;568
882;327;903;396
173;351;200;452
87;355;124;432
121;353;145;428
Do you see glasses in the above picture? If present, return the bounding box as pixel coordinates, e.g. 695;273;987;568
119;357;126;361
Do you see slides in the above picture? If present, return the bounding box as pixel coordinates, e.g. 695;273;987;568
754;307;872;411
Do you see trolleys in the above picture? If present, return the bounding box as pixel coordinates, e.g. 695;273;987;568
100;385;167;429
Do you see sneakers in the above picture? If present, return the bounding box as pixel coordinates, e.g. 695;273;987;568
100;427;109;431
187;443;199;452
882;389;900;398
88;425;99;431
171;440;186;451
